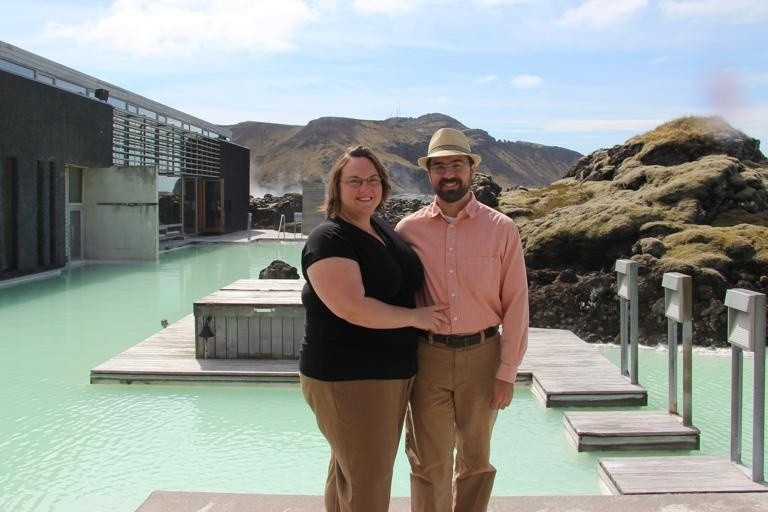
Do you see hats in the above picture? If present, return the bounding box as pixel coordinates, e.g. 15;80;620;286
418;127;482;171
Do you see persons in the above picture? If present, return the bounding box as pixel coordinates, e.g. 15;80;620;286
298;144;452;511
393;126;530;512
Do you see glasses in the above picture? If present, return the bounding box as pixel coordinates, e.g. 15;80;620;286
344;175;380;187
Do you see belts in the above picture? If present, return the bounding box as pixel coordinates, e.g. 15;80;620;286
419;325;498;349
